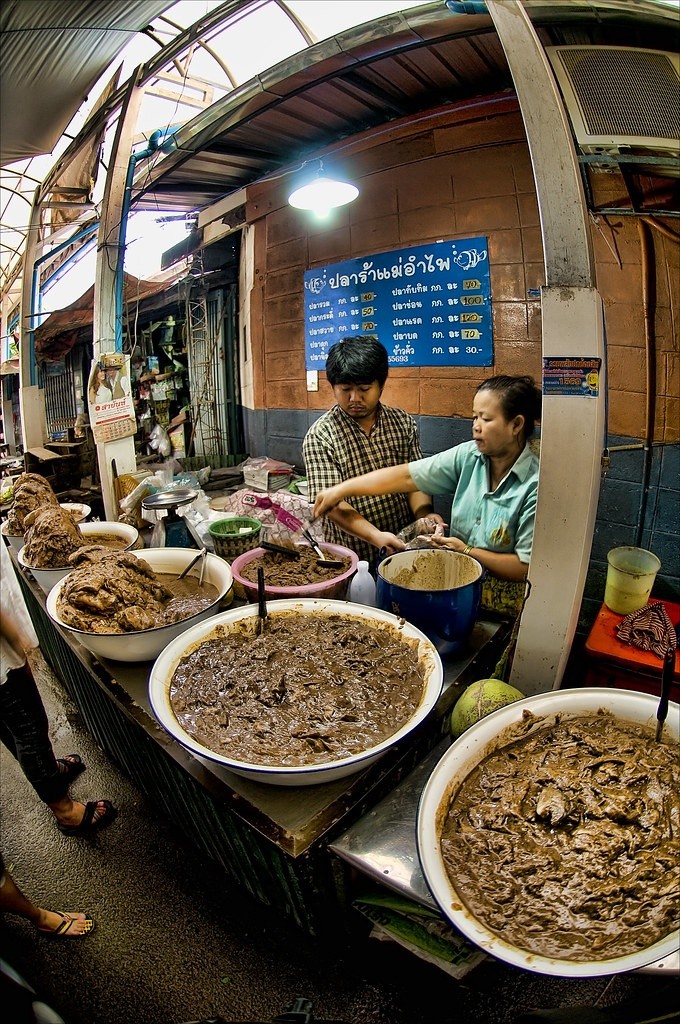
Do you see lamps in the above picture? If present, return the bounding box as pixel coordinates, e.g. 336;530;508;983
289;158;360;211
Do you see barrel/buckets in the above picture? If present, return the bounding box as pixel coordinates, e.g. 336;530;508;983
208;517;262;566
604;545;661;615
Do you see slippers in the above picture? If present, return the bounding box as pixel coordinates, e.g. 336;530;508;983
36;909;95;941
57;754;82;778
56;799;114;832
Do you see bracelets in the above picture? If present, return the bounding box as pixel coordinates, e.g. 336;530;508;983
463;546;473;554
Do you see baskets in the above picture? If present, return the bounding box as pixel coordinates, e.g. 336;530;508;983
208;517;261;565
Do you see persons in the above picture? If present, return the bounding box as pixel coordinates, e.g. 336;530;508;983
301;335;448;563
311;376;541;618
0;537;112;831
0;853;95;938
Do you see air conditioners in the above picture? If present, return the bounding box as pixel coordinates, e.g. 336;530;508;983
545;45;680;176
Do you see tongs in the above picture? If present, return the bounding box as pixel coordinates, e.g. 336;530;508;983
177;548;207;588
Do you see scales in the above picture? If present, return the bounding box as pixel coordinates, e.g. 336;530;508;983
139;491;199;549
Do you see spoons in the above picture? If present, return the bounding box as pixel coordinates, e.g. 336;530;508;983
303;530;344;568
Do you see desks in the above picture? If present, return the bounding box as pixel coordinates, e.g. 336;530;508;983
324;731;680;983
6;527;503;939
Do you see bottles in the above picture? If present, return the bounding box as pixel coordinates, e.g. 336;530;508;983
349;560;377;607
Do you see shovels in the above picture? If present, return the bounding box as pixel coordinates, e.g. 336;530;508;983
300;525;345;568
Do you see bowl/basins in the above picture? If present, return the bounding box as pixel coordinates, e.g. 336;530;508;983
231;540;359;604
46;546;236;662
414;686;680;978
146;597;444;787
295;480;310;496
0;502;91;554
374;546;483;655
18;522;140;596
